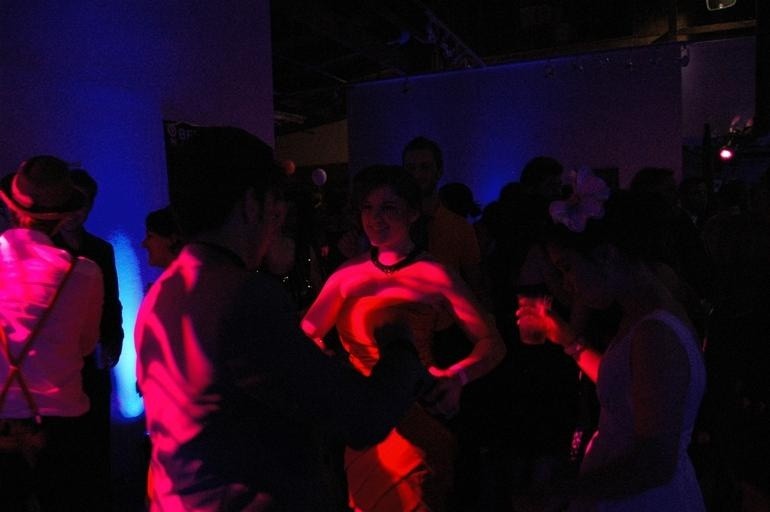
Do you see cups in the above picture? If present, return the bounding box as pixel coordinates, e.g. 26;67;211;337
515;290;553;345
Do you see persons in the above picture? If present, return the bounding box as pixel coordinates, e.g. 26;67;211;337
1;157;125;511
137;122;768;512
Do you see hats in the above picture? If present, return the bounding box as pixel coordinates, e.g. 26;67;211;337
0;155;96;221
